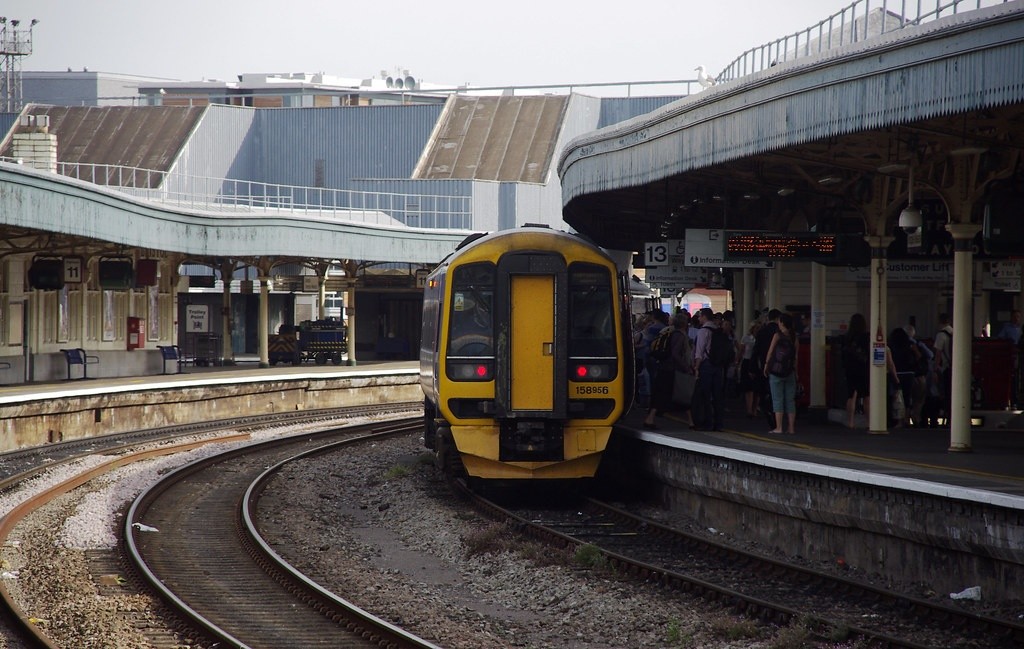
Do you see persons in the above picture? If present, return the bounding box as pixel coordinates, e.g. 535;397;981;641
630;302;1024;436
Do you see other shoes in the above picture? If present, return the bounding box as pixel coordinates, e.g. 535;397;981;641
643;421;662;430
1011;403;1018;410
689;424;696;429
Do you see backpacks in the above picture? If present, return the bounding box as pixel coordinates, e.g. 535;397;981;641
767;330;797;378
935;329;952;359
694;325;734;366
914;338;930;377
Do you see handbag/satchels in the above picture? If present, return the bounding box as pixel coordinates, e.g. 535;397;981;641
725;357;745;395
644;347;676;389
674;366;700;407
892;382;906;420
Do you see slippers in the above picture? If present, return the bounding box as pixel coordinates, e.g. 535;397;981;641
768;429;784;435
784;430;796;435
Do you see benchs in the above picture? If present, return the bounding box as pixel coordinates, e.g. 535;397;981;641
156;345;192;375
60;348;100;381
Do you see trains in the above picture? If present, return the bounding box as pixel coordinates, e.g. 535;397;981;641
419;223;663;479
